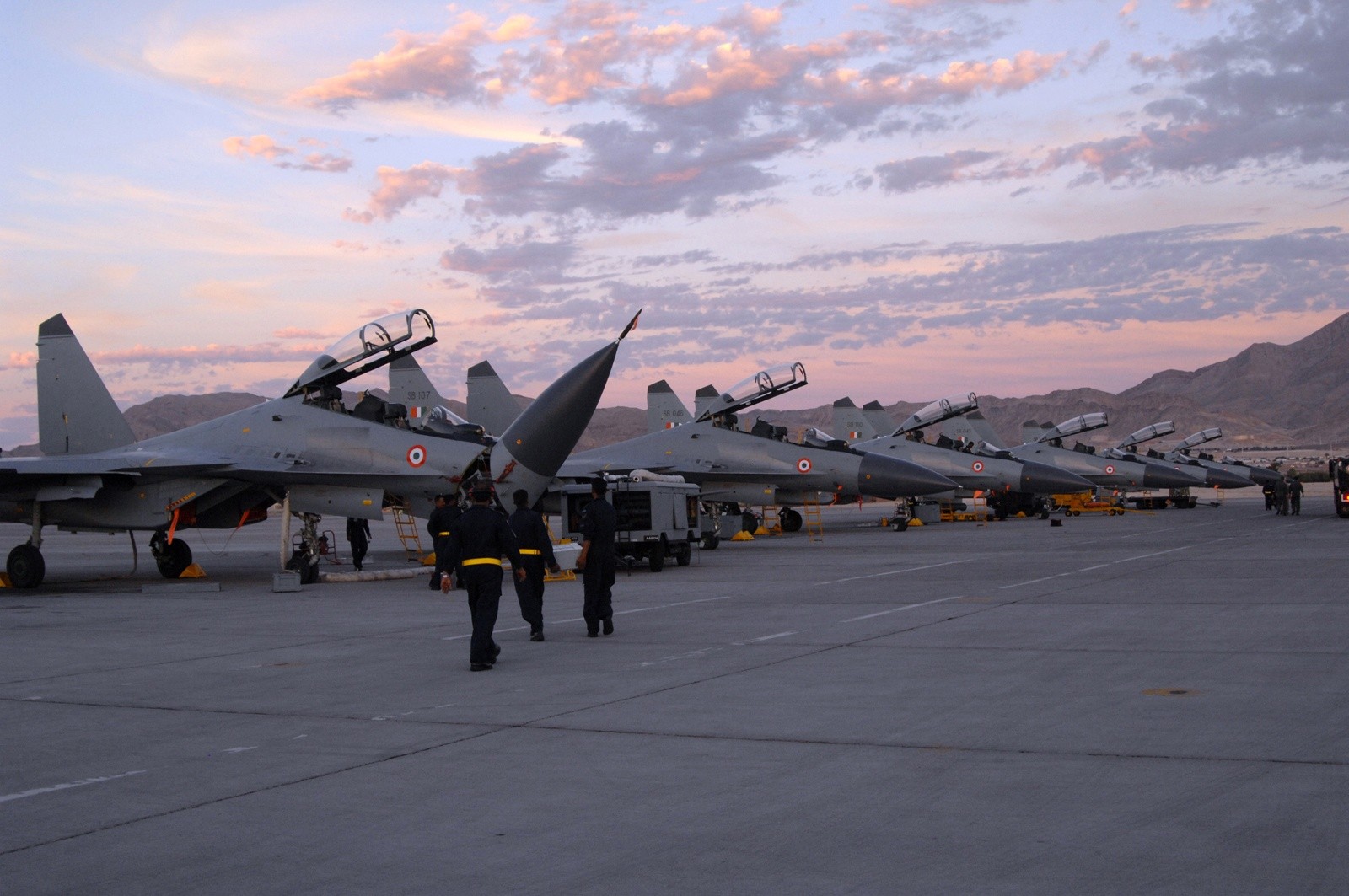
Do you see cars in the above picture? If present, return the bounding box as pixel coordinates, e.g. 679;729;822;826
1328;454;1349;518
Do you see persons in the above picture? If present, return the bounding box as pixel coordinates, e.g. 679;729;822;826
346;517;372;571
1262;475;1305;516
429;492;467;591
441;485;526;673
508;489;560;642
964;441;974;455
575;477;616;638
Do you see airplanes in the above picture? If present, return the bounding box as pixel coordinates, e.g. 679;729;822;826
1094;421;1253;492
977;415;1204;524
800;394;1099;522
1165;425;1291;490
2;302;644;592
551;361;964;542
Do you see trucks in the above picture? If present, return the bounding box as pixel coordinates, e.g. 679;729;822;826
559;472;701;572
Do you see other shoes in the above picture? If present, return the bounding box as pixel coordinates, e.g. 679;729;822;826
603;618;614;635
471;637;501;671
586;631;597;637
530;630;546;641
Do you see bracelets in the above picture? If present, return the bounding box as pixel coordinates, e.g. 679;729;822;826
442;573;450;578
